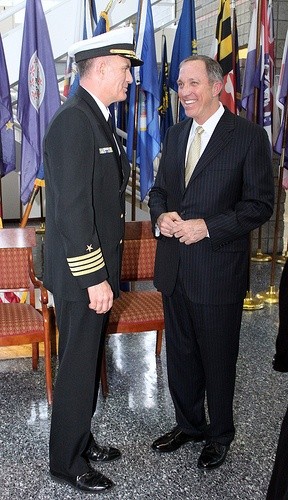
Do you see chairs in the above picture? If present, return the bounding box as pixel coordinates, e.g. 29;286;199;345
98;219;168;400
0;227;53;405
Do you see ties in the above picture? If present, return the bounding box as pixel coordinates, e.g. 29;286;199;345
185;126;204;188
107;114;120;159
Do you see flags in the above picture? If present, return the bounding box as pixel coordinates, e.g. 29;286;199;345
212;1;243;117
274;30;288;189
0;34;17;231
64;0;169;203
17;0;62;205
167;0;197;128
241;0;276;155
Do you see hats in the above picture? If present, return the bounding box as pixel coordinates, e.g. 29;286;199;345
68;27;144;67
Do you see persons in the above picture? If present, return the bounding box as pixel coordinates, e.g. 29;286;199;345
149;54;277;470
43;26;144;493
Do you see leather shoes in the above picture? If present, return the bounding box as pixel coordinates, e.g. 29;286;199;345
49;464;115;493
197;441;229;470
151;428;204;452
85;443;122;462
